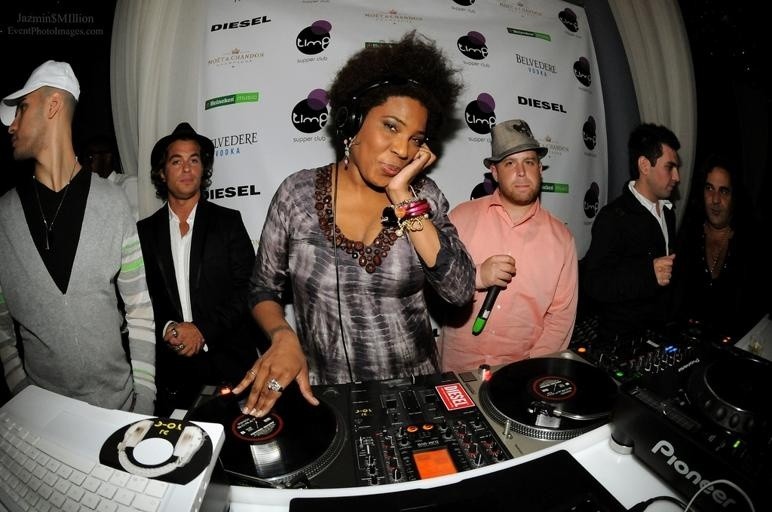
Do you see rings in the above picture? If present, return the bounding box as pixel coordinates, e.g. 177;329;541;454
173;345;183;353
249;368;257;377
270;379;284;393
264;380;272;391
172;328;177;337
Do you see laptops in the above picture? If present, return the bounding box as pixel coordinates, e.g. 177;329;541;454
0;384;226;512
289;449;629;512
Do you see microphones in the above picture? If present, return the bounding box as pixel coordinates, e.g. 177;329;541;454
472;285;500;335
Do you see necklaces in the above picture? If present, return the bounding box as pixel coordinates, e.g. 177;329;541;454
706;230;731;275
30;155;78;251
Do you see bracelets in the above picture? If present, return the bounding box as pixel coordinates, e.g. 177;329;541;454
266;324;294;340
387;196;430;236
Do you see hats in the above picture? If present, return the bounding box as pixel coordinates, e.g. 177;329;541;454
0;58;82;126
149;122;215;171
483;119;549;170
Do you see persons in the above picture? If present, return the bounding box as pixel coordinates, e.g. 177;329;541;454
225;31;474;421
578;122;680;341
136;122;257;409
677;159;756;343
0;59;158;417
433;117;578;375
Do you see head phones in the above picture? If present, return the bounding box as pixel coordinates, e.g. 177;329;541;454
333;69;437;142
